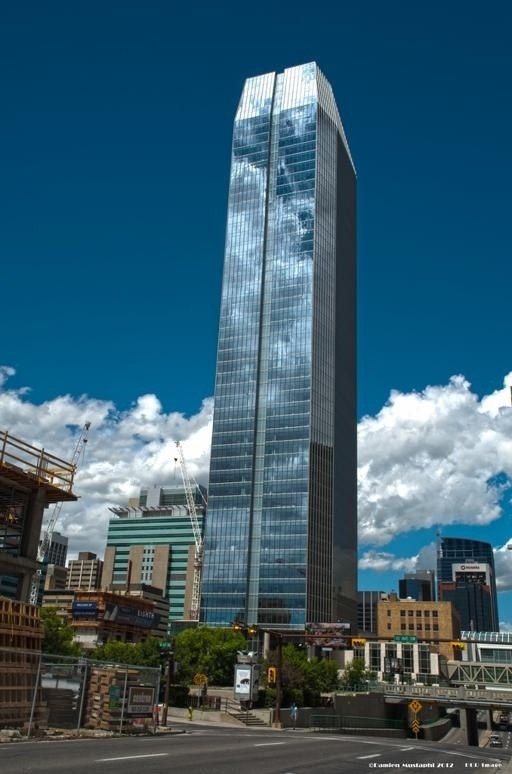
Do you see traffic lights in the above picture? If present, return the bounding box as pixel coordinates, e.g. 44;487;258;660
267;668;276;683
248;627;257;636
450;640;467;651
351;638;367;647
233;624;242;633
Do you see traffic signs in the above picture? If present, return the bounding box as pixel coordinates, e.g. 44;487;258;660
394;634;418;643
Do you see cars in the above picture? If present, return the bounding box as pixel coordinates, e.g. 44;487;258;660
488;709;509;748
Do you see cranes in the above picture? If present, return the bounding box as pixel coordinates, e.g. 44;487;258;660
169;436;210;622
27;420;95;605
